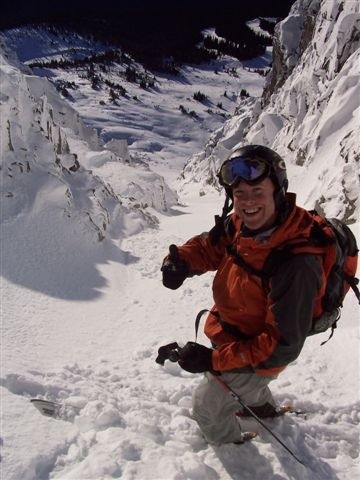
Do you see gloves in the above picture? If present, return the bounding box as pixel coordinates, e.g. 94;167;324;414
177;342;222;376
160;244;190;290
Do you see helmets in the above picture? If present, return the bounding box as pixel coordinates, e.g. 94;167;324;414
214;144;288;219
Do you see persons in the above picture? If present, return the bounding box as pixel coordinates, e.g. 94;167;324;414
162;144;329;446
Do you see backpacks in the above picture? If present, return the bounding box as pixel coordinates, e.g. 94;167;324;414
195;200;360;346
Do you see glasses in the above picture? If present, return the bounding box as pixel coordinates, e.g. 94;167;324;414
218;155;273;189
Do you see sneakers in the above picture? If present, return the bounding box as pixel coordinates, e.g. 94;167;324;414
235;401;277;419
